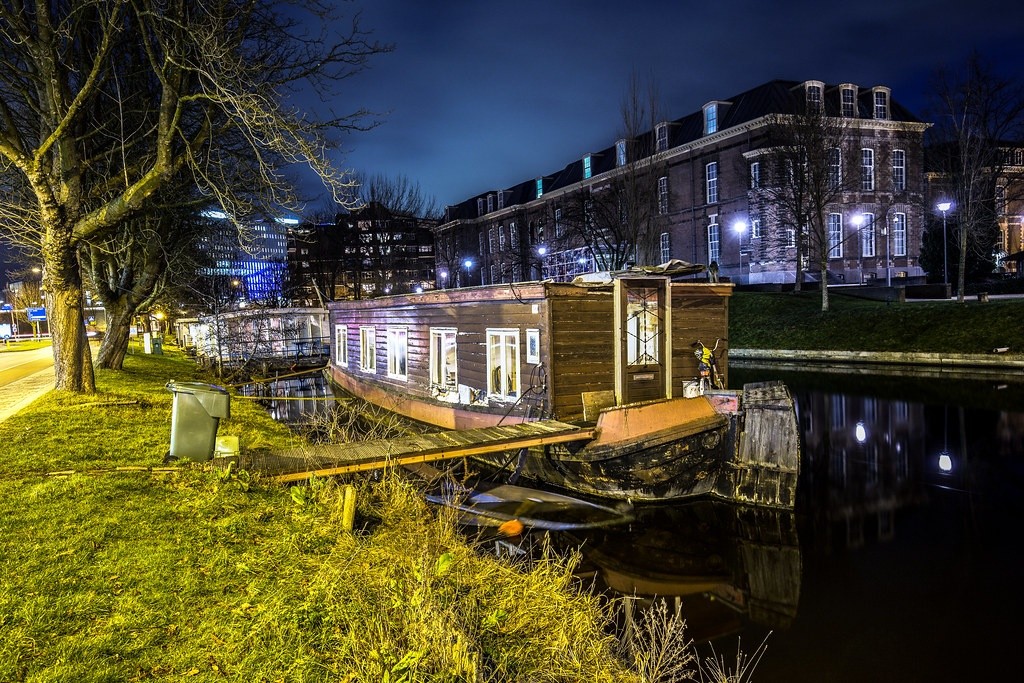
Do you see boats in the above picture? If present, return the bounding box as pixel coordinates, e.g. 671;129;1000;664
320;258;804;512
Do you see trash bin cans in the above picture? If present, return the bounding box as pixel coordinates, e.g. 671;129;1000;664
164;383;230;464
153;339;162;355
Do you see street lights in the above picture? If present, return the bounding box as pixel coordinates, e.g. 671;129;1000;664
852;214;864;264
538;247;546;280
32;268;40;296
937;202;952;283
735;221;746;273
441;272;447;289
465;260;472;286
